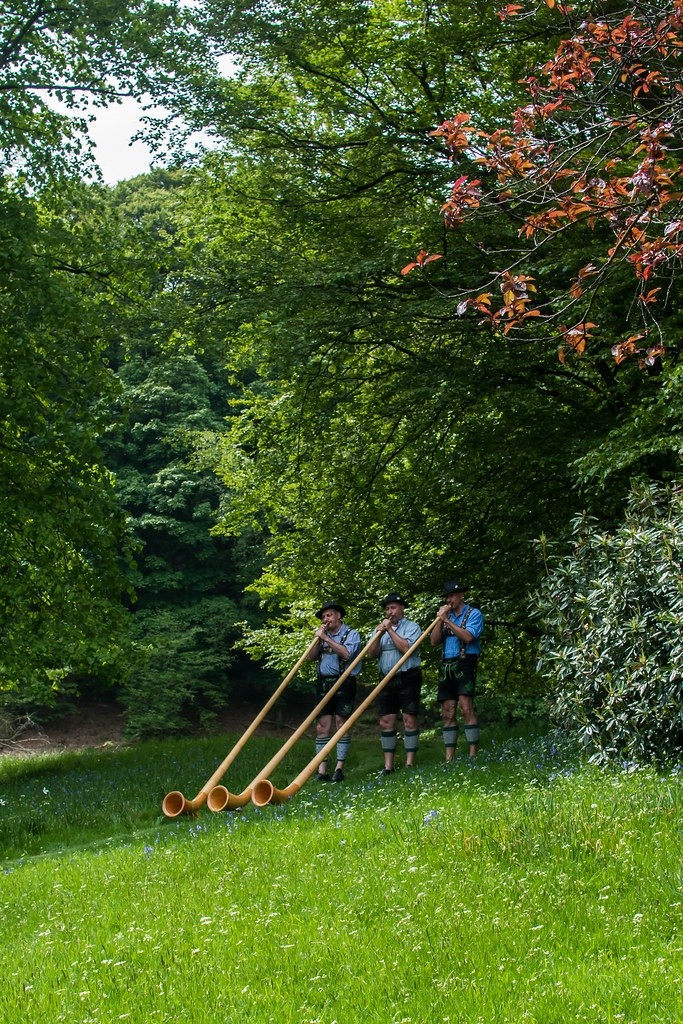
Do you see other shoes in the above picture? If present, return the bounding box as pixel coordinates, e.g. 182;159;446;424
385;767;396;774
316;773;330;781
332;769;344;781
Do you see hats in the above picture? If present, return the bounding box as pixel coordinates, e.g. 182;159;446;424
379;593;410;609
439;581;468;597
315;603;345;620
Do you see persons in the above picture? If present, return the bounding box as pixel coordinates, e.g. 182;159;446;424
430;581;486;765
305;600;362;781
368;593;423;775
